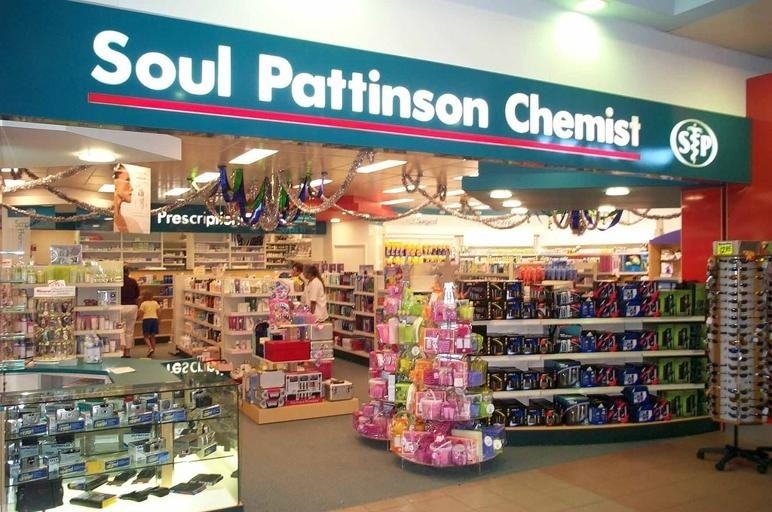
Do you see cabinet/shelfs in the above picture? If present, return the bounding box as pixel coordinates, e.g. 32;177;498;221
0;230;772;512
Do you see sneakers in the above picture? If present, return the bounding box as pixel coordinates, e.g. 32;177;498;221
147;347;154;358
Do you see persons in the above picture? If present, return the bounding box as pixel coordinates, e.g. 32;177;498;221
278;262;305;302
121;265;140;358
301;265;328;324
135;288;161;356
112;162;144;233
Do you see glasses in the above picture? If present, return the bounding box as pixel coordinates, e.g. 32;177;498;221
703;257;772;423
